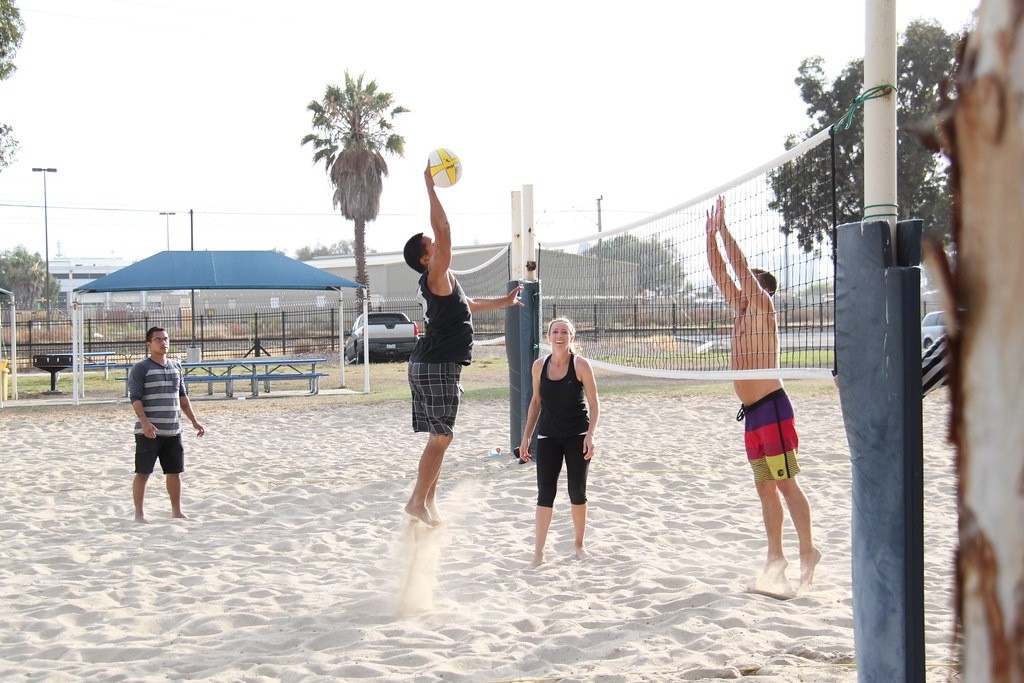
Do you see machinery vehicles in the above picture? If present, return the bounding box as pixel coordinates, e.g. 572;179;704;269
32;297;51;322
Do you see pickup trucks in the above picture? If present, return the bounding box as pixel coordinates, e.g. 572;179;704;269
342;312;420;365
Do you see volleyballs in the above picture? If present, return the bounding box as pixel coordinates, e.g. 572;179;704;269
428;148;462;187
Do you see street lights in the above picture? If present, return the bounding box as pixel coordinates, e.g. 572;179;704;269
159;212;176;252
32;167;58;332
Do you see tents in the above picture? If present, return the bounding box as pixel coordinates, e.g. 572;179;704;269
71;250;369;408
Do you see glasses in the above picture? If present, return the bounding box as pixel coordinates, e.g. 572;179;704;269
150;337;169;344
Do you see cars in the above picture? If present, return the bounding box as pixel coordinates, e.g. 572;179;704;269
920;309;946;350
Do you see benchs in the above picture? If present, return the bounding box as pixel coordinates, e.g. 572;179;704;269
55;363;119;368
115;372;332;380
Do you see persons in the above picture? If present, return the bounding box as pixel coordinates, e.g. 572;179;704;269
403;159;524;529
706;195;821;588
520;316;602;569
127;326;205;522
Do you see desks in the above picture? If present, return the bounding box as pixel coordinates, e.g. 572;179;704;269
47;352;116;380
115;358;326;393
